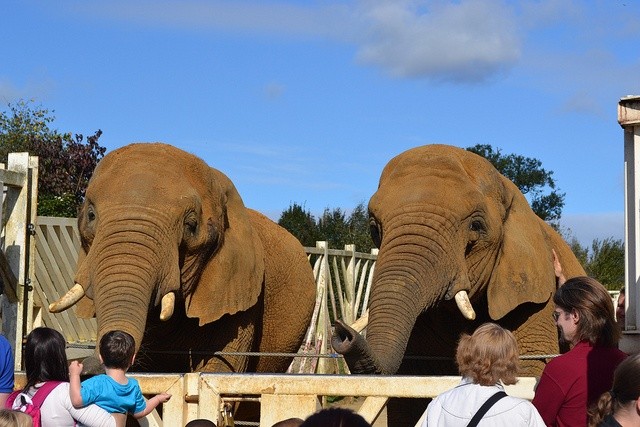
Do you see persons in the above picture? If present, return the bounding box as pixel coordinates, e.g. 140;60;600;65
587;353;640;427
616;286;625;329
68;330;173;418
531;276;629;427
415;322;548;427
12;328;128;427
0;410;31;427
299;407;372;427
0;334;14;410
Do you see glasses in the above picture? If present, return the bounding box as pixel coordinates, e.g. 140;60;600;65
552;311;570;322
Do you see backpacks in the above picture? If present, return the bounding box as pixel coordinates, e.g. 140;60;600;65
7;379;77;427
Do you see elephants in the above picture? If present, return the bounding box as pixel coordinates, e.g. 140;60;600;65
48;141;317;418
330;143;589;426
